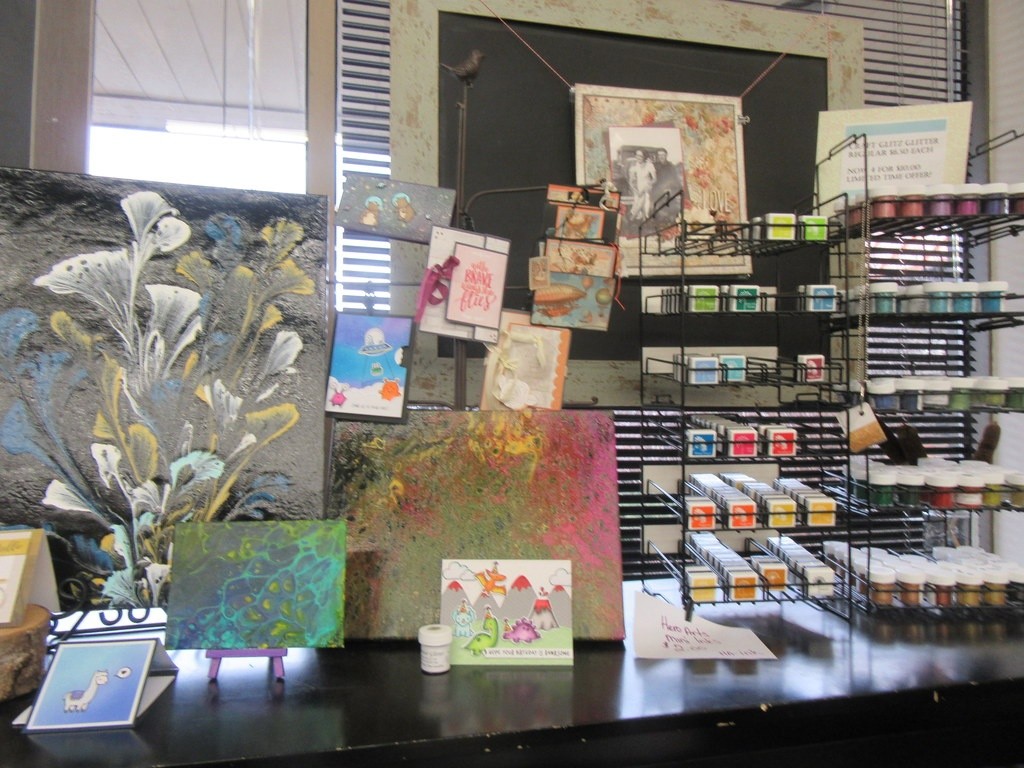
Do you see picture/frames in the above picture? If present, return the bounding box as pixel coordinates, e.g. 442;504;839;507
572;83;754;278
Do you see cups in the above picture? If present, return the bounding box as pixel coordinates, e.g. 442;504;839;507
822;176;1024;606
417;624;453;674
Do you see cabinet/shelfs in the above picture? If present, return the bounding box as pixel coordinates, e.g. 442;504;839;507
639;181;856;629
808;125;1024;621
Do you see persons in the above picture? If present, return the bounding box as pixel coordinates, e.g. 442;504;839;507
627;146;657;222
654;148;682;193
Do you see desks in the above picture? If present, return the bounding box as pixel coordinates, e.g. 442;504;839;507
0;554;1024;768
